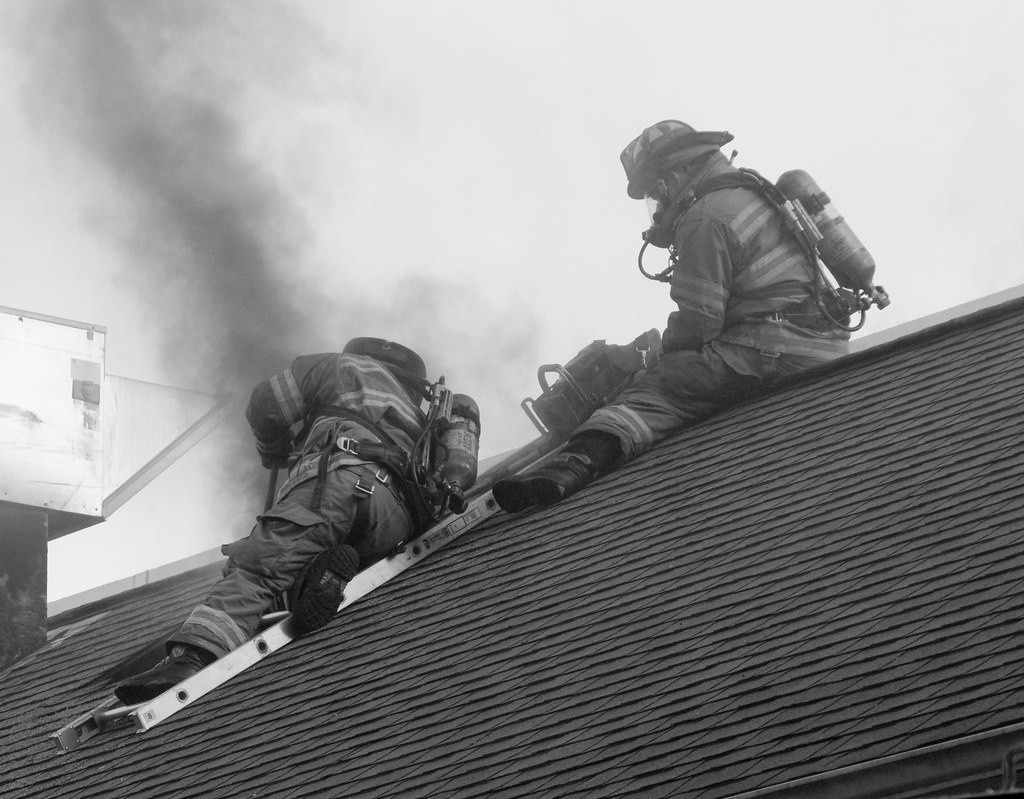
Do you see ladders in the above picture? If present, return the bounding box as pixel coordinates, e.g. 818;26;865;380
48;424;569;754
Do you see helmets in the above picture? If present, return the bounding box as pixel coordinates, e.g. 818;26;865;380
619;119;733;200
343;336;427;407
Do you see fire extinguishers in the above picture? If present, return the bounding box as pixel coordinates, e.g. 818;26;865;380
776;170;889;312
435;390;481;508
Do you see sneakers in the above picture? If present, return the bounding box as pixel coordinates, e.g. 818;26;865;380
489;451;598;513
289;544;361;636
114;644;209;706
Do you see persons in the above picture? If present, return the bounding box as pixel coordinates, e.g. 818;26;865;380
115;336;427;705
493;120;851;514
